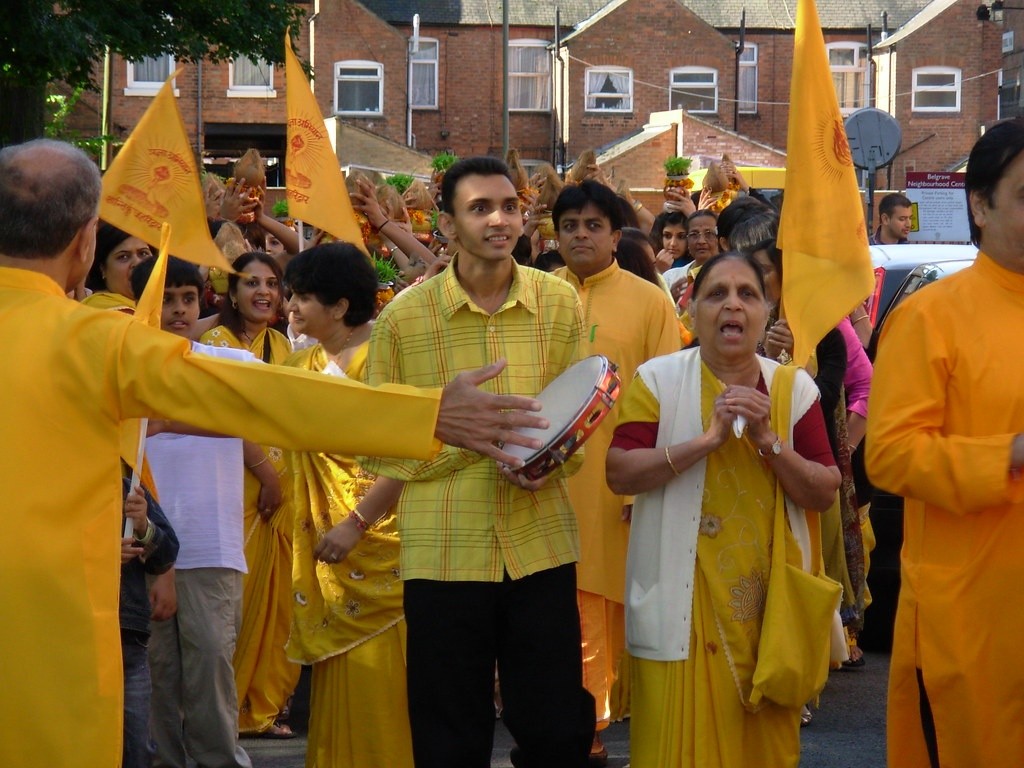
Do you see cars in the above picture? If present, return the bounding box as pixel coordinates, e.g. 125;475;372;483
863;244;978;329
852;260;974;659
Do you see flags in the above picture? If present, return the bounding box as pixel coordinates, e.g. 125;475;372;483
775;0;877;368
282;34;373;262
100;82;240;274
118;224;171;502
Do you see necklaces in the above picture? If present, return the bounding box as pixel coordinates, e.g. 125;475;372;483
242;328;254;344
334;328;354;365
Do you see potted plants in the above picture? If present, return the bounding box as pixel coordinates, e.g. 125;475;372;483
431;149;460;189
664;155;693;180
373;251;401;289
272;200;289;222
430;208;448;244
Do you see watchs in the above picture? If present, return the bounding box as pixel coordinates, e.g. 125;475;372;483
759;436;783;460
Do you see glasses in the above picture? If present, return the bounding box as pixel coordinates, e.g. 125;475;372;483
685;231;718;241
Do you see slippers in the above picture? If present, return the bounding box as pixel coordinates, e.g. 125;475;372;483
846;643;865;667
238;719;297;740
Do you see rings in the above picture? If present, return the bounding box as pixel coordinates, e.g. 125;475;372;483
332;553;337;561
265;508;271;513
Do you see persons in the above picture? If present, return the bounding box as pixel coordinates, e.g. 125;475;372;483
0;137;913;768
863;120;1024;768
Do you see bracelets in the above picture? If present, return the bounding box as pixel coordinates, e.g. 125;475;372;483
247;456;268;470
348;511;369;534
353;508;370;528
377;220;389;231
848;443;858;451
665;447;680;479
632;198;642;214
389;245;399;254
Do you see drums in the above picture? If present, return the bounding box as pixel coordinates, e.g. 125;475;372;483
497;354;622;482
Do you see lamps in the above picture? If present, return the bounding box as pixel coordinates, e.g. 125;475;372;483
976;0;1024;21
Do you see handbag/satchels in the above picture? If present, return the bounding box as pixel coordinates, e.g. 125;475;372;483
747;562;845;711
